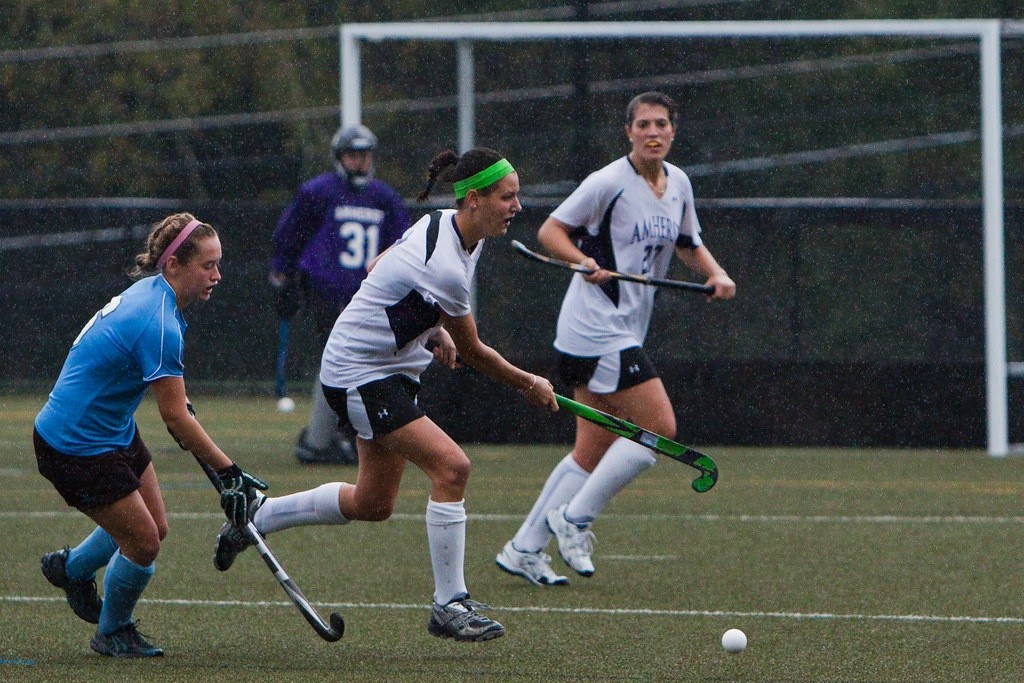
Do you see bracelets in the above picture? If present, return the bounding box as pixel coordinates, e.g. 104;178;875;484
521;369;536;396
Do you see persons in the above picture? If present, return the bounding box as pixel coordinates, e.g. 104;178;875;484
212;148;560;641
267;124;411;462
32;211;269;659
496;94;737;586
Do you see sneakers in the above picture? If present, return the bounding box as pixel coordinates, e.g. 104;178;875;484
546;503;598;574
496;540;569;588
90;619;162;658
213;487;268;571
428;593;506;642
41;544;104;624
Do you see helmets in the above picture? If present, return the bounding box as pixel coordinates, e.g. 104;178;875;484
331;124;378;150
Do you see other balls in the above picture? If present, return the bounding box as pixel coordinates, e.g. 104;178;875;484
278;397;295;413
720;628;748;654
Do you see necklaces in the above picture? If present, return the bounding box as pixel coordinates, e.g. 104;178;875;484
641;174;664;195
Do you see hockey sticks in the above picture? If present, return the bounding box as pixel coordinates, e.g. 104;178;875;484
188;447;348;643
275;320;291;396
424;340;720;496
510;237;714;296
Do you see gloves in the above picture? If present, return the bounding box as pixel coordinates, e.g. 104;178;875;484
272;279;302;319
216;461;268;527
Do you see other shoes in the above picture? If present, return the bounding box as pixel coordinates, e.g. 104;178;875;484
295;429;350;464
